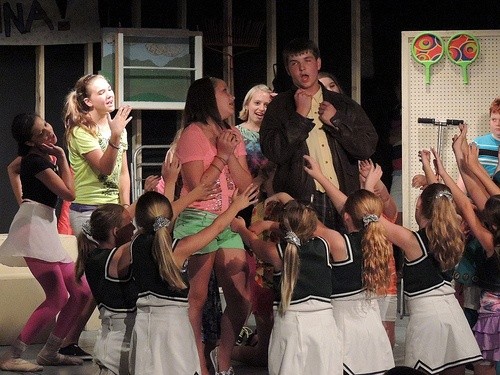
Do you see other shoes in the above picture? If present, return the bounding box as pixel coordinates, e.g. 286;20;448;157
59;343;93;360
210;346;234;375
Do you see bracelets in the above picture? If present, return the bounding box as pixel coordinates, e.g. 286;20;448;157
210;155;228;173
108;139;120;150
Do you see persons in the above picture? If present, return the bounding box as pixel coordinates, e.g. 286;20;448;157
316;71;347;95
0;113;92;372
232;82;283;345
175;76;252;374
57;73;131;361
76;96;499;375
259;38;379;230
